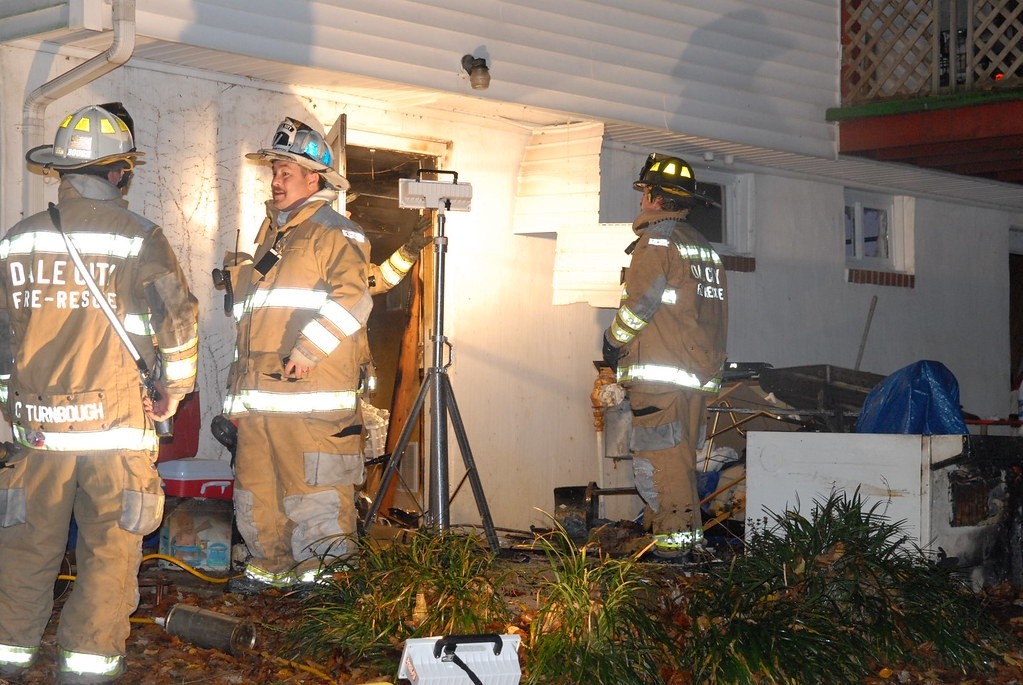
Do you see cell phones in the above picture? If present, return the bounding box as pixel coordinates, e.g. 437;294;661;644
253;249;283;278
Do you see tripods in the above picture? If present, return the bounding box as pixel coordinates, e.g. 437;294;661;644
359;206;501;564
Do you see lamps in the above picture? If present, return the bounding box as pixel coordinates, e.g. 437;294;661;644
461;54;491;90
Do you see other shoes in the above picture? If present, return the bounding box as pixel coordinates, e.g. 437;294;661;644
59;661;128;684
1;654;42;680
640;547;704;566
300;575;347;602
224;576;293;597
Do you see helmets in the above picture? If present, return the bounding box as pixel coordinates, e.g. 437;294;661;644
24;106;145;170
633;158;696;198
245;117;350;190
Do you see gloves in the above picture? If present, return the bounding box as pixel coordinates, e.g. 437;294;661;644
403;215;433;256
602;330;619;373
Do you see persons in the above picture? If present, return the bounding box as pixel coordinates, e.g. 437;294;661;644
221;125;432;592
603;158;728;564
0;104;201;685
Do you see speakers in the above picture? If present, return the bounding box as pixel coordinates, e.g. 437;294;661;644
552;486;602;539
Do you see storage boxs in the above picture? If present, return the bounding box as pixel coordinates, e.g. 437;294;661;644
158;497;234;572
